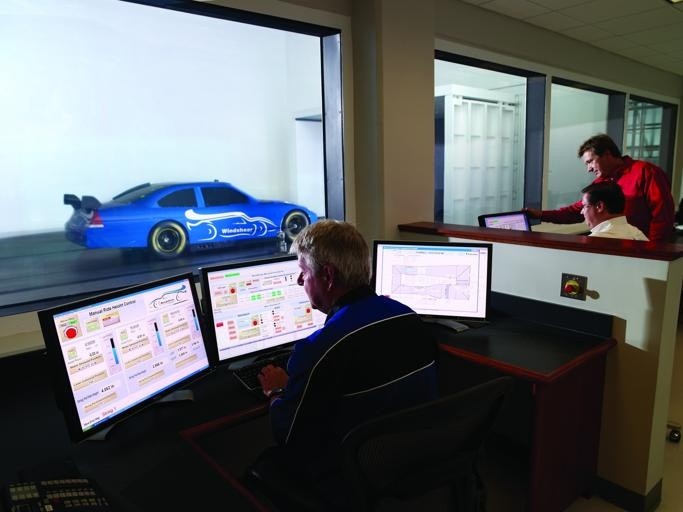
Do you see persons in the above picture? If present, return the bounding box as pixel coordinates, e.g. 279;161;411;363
522;134;675;241
580;180;649;242
246;218;440;512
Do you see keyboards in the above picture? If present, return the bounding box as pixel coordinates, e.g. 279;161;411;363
232;352;292;392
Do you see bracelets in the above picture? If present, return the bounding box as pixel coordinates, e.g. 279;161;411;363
268;387;286;399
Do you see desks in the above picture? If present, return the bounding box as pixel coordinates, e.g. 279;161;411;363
0;309;616;512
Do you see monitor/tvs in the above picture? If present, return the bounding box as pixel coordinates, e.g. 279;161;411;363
37;271;216;443
198;254;328;371
478;210;532;232
370;240;492;332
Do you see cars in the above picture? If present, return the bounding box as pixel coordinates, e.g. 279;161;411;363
62;179;321;260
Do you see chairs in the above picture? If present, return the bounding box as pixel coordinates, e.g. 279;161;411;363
294;377;505;512
